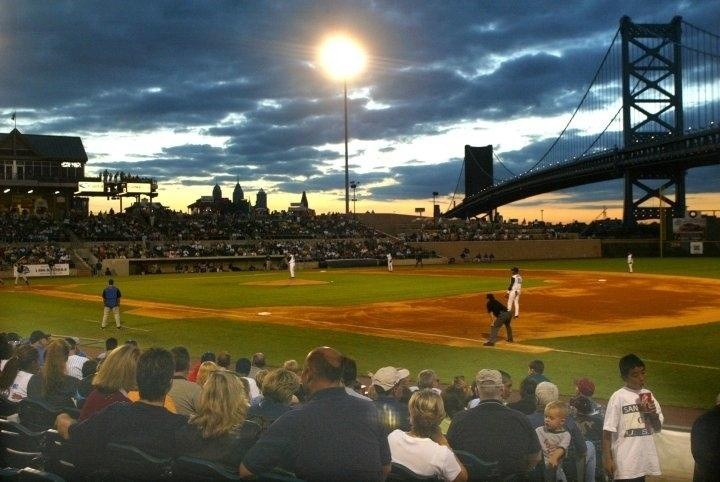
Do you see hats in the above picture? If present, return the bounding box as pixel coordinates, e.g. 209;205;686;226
475;367;504;391
371;365;411;393
571;378;596;398
65;336;81;350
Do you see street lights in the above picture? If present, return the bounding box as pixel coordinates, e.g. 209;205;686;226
314;31;373;216
349;179;361;213
431;191;439;229
541;209;545;220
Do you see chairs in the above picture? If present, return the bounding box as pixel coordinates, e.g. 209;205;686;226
0;385;308;481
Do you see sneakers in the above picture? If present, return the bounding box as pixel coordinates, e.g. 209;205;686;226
29;330;51;344
505;339;513;343
483;341;494;346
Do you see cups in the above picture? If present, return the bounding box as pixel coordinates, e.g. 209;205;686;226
638;391;652;413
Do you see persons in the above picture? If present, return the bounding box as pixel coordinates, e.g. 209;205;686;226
690;392;720;481
100;279;122;329
504;267;523;319
1;207;665;286
483;294;514;347
1;330;664;481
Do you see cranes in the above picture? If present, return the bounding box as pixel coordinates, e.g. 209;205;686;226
594;205;607;220
